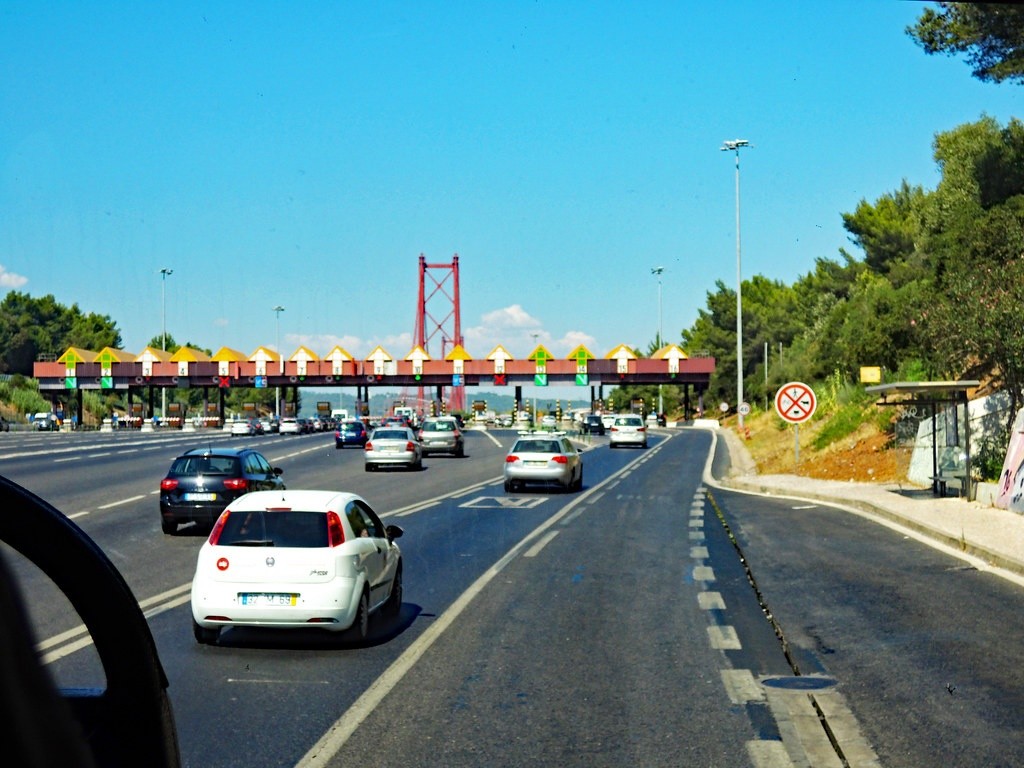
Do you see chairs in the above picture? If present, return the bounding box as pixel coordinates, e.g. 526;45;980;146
524;443;536;451
196;459;211;471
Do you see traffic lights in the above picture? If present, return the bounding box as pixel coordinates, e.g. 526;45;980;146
217;372;678;385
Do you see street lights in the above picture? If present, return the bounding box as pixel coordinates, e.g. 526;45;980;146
651;266;665;414
720;138;751;424
273;305;286;415
159;268;174;418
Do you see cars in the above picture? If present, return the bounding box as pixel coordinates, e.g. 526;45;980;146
334;422;370;448
651;413;667;427
231;419;256;437
502;434;585;492
541;415;558;430
0;416;10;432
245;407;465;437
484;411;530;429
364;426;423;472
190;490;404;646
32;418;60;432
278;417;301;435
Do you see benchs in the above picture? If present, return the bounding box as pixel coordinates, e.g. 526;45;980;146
929;476;966;497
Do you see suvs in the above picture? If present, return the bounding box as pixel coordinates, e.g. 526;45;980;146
0;473;182;768
579;415;605;437
160;446;286;536
609;413;649;449
416;416;466;458
